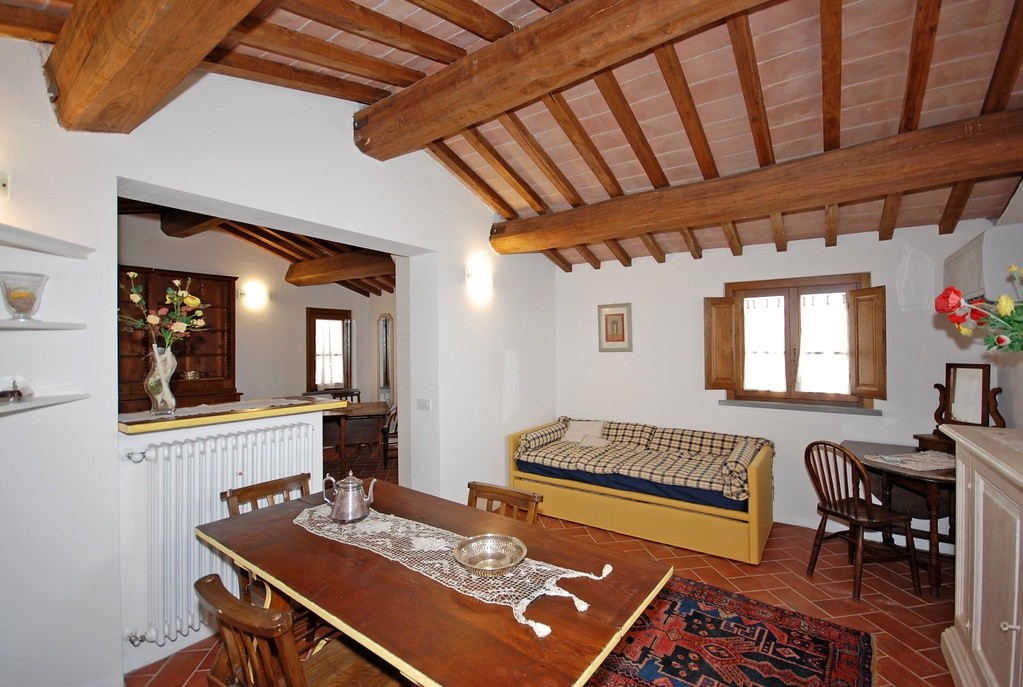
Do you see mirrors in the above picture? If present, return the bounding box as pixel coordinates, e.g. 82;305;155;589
913;363;1006;456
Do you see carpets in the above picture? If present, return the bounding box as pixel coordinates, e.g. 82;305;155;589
582;576;880;687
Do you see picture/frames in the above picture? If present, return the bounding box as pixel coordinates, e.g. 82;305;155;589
598;303;632;352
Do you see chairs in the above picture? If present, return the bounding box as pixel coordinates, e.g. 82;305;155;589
194;574;412;687
805;440;921;602
381;406;398;471
466;481;544;524
220;473;339;655
331;390;373;456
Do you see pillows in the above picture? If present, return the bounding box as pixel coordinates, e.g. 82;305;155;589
562;421;605;444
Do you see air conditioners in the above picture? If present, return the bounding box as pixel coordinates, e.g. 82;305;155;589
943;223;1019;305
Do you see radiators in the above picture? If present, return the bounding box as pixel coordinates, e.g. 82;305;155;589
125;422;314;648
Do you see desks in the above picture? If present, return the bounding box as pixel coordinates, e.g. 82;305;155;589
323;401;391;472
839;440;956;598
195;478;674;687
321;409;348;479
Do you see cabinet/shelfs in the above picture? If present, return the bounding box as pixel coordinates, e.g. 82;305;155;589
938;424;1023;687
117;264;244;414
0;217;97;419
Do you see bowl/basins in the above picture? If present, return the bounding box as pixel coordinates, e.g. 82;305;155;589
0;271;49;321
453;534;528;577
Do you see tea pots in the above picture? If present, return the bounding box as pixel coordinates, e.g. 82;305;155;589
323;470;377;520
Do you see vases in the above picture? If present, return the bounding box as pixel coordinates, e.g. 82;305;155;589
144;348;177;416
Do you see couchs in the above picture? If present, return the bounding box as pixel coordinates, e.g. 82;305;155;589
507;416;776;565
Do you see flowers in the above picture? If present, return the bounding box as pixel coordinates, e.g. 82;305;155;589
117;271;211;408
934;265;1023;354
